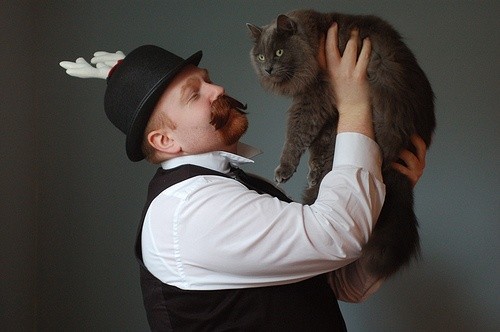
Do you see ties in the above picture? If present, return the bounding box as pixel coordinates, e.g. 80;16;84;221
230;168;293;203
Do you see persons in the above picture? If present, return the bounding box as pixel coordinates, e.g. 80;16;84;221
106;22;426;332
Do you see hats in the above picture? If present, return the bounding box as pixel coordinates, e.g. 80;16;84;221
59;45;204;162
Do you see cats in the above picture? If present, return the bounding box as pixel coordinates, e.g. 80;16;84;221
246;8;439;283
